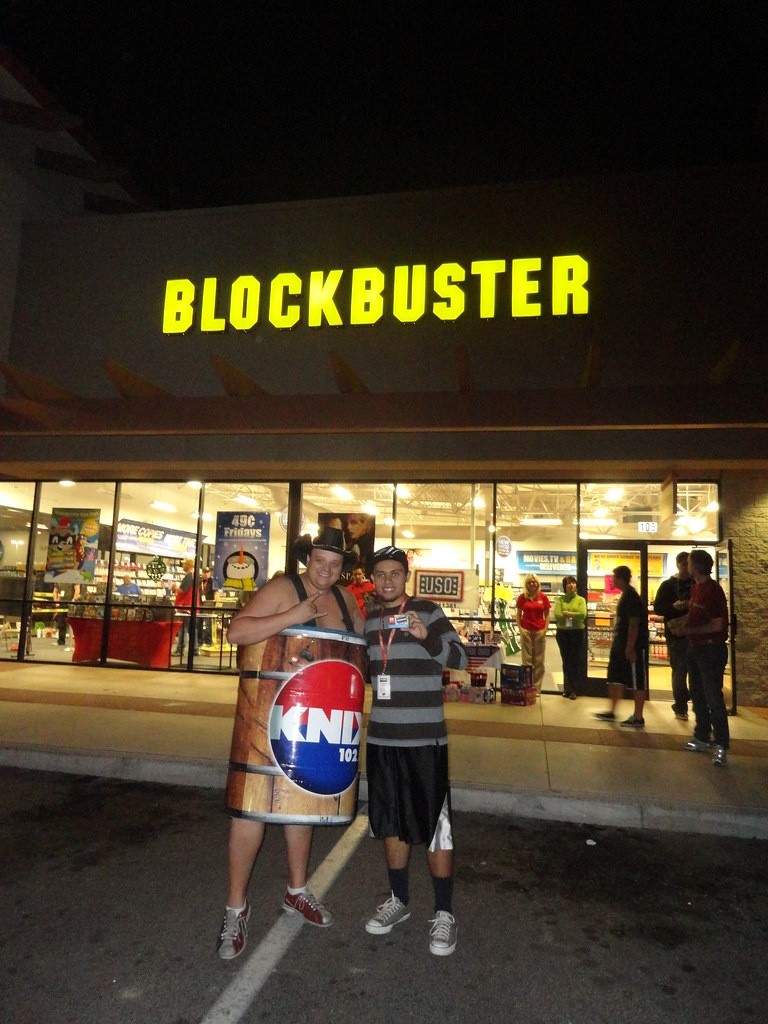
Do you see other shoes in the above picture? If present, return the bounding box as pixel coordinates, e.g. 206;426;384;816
671;703;689;720
595;712;617;721
712;745;728;768
619;715;645;727
562;691;569;698
679;739;711;752
569;692;577;699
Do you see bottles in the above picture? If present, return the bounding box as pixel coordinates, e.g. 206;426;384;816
565;615;573;628
441;668;495;704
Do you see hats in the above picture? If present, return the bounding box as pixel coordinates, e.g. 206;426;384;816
364;544;408;576
291;526;360;576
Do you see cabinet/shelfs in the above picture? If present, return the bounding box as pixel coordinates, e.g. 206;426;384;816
90;559;185;596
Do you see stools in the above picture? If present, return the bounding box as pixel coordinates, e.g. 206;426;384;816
3;628;21;650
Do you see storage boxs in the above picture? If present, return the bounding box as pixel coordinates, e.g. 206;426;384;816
501;687;537;707
499;662;534;689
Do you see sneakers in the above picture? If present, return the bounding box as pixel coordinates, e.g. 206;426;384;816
280;886;336;928
216;898;251;960
365;889;411;935
426;909;459;957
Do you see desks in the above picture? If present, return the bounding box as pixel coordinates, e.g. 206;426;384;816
67;617;183;669
174;612;232;670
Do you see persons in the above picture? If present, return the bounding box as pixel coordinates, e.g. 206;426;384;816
318;512;375;581
360;545;469;957
50;583;87;651
594;565;649;728
347;567;375;619
163;580;178;595
171;559;214;655
516;573;552;696
215;526;366;960
666;550;729;767
116;575;141;594
554;576;588;699
654;553;695;721
